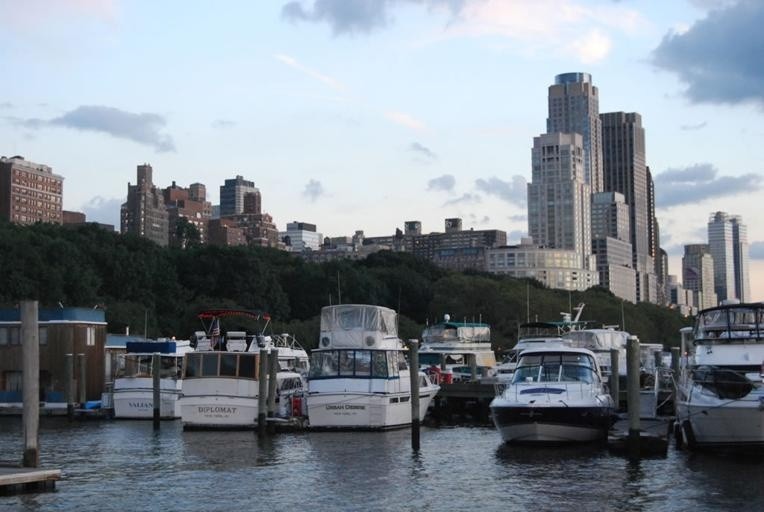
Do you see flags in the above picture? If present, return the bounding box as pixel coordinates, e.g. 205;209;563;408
210;318;219;346
